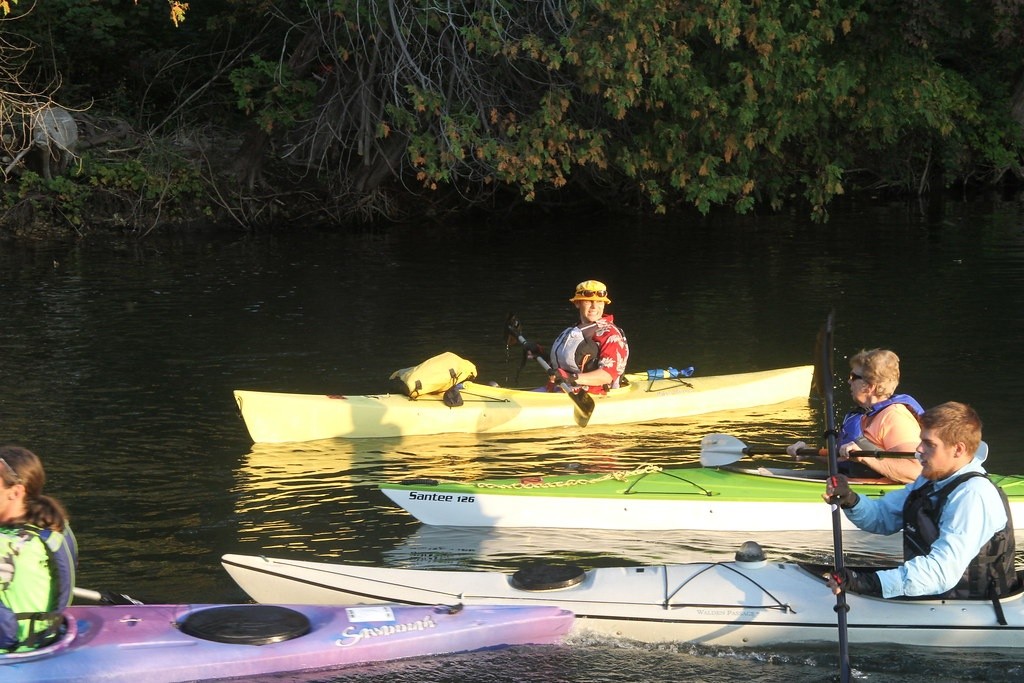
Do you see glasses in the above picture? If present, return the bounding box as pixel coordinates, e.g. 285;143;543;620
577;290;607;297
848;372;862;382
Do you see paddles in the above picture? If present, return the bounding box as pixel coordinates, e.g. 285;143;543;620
701;432;986;469
816;309;852;683
504;312;595;422
74;584;143;606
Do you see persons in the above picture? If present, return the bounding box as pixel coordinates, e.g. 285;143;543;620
786;348;926;485
522;280;630;395
821;401;1016;602
0;444;79;655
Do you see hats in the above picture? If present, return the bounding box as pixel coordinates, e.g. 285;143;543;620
569;280;611;304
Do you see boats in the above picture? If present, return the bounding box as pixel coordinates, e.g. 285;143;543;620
233;364;816;443
385;525;1023;569
379;465;1024;532
0;606;575;681
220;555;1024;650
